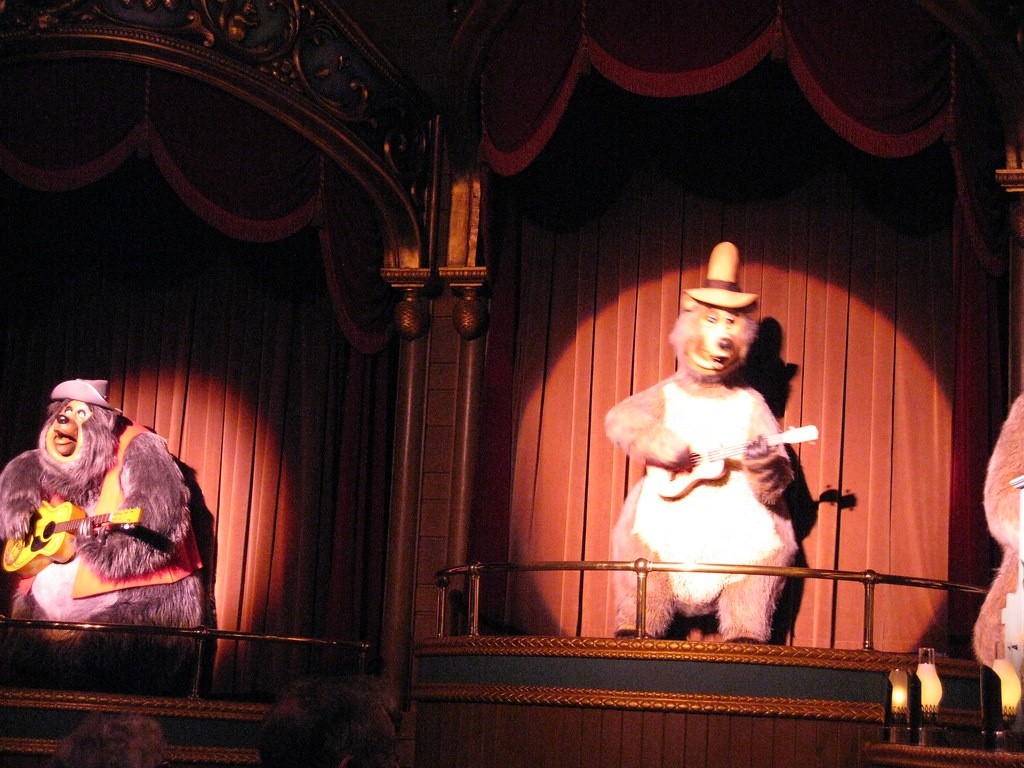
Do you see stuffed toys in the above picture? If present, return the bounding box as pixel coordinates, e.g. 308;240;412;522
0;378;202;695
973;392;1024;684
606;242;798;642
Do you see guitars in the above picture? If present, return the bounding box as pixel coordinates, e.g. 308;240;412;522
646;423;819;500
2;500;142;579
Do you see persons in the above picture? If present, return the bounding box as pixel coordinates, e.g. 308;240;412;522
43;714;167;768
257;677;399;768
